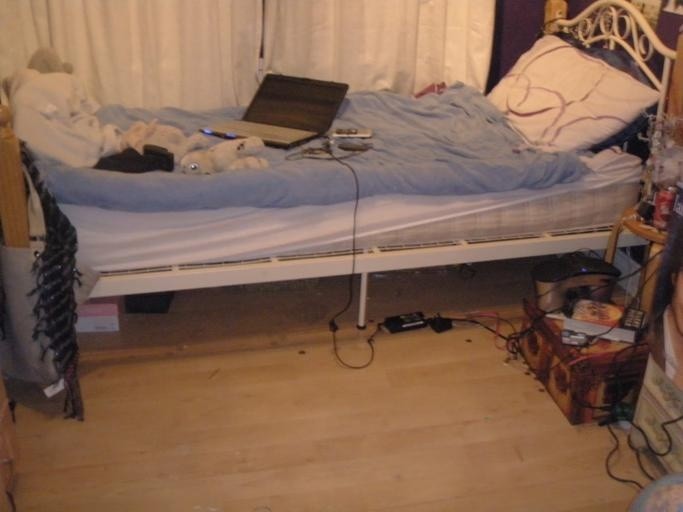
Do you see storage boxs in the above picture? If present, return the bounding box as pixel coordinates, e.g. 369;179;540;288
74;299;123;333
518;297;649;425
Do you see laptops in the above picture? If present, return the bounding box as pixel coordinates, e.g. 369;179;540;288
200;73;349;149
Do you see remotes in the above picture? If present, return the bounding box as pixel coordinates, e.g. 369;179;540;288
623;308;647;343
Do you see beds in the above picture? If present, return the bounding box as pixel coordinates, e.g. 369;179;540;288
0;0;683;346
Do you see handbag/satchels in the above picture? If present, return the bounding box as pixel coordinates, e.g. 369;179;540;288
0;245;101;385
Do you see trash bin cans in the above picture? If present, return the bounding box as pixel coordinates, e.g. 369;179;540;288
628;475;683;512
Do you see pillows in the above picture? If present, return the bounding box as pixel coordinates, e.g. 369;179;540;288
484;34;658;156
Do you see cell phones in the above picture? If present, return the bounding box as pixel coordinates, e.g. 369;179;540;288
332;128;373;139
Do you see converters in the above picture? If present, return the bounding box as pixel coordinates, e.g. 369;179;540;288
382;311;426;334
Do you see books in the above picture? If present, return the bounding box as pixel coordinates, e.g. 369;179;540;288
562;298;646;346
